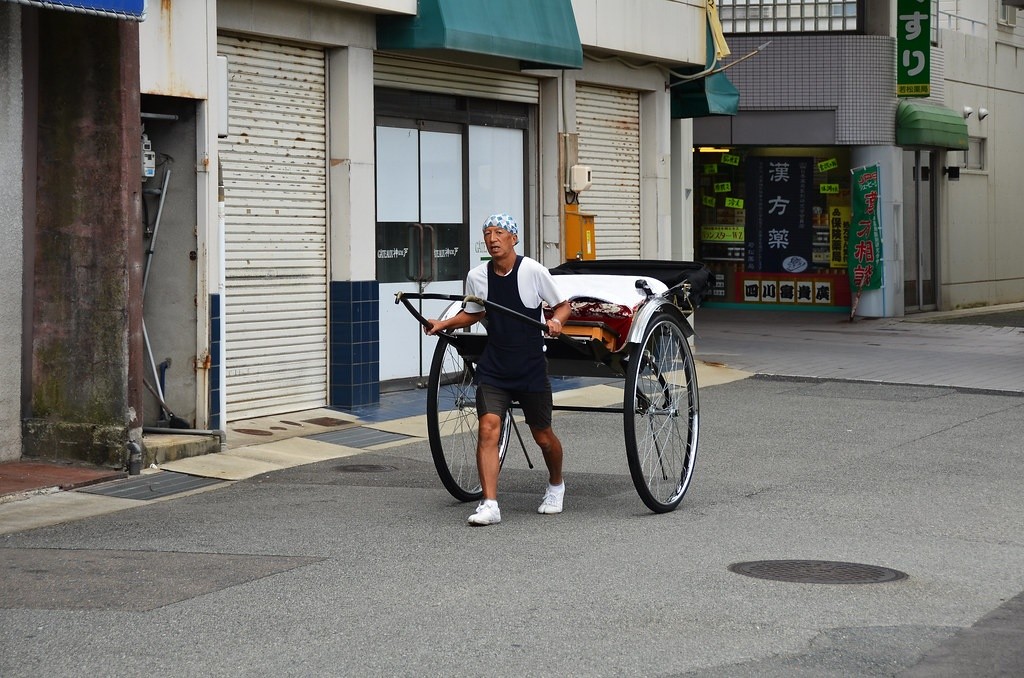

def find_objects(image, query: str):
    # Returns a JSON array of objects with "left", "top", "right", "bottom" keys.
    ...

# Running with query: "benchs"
[{"left": 539, "top": 274, "right": 670, "bottom": 351}]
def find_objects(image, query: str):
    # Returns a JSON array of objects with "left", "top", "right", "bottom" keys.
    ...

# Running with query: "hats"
[{"left": 482, "top": 213, "right": 518, "bottom": 234}]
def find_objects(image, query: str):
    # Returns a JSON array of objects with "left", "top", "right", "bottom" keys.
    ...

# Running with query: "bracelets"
[{"left": 551, "top": 317, "right": 562, "bottom": 325}]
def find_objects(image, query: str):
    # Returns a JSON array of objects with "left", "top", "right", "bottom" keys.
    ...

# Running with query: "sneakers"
[
  {"left": 537, "top": 478, "right": 565, "bottom": 513},
  {"left": 468, "top": 500, "right": 501, "bottom": 525}
]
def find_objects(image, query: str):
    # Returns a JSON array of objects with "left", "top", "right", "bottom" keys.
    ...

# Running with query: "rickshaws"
[{"left": 394, "top": 258, "right": 712, "bottom": 514}]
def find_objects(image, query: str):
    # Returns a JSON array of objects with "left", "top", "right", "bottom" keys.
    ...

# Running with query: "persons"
[{"left": 424, "top": 213, "right": 572, "bottom": 526}]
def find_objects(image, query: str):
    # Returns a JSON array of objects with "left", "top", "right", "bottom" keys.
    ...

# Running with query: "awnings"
[
  {"left": 896, "top": 98, "right": 970, "bottom": 152},
  {"left": 671, "top": 0, "right": 741, "bottom": 118},
  {"left": 376, "top": 0, "right": 585, "bottom": 71}
]
